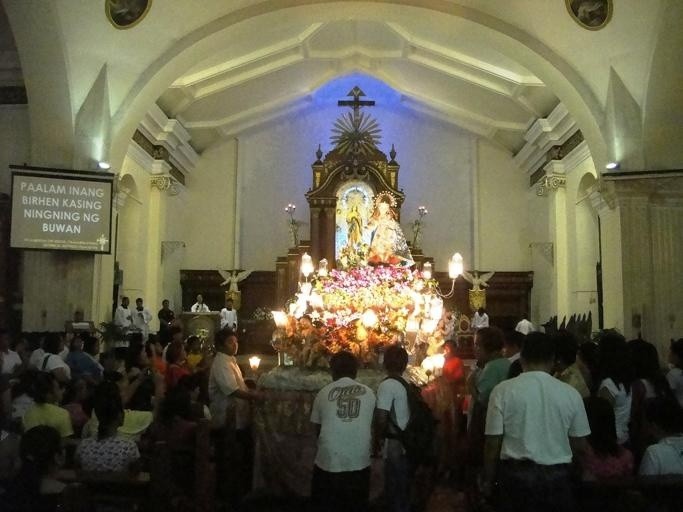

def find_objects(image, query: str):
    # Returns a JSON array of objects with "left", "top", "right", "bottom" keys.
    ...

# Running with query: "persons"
[
  {"left": 443, "top": 307, "right": 682, "bottom": 512},
  {"left": 0, "top": 294, "right": 267, "bottom": 512},
  {"left": 376, "top": 346, "right": 412, "bottom": 512},
  {"left": 310, "top": 350, "right": 377, "bottom": 512},
  {"left": 462, "top": 270, "right": 495, "bottom": 290},
  {"left": 346, "top": 205, "right": 363, "bottom": 245},
  {"left": 218, "top": 267, "right": 251, "bottom": 291}
]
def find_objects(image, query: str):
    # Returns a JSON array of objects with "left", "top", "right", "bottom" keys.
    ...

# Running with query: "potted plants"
[{"left": 96, "top": 322, "right": 124, "bottom": 352}]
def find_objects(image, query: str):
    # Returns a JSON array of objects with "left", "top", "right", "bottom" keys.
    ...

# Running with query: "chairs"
[{"left": 62, "top": 487, "right": 143, "bottom": 511}]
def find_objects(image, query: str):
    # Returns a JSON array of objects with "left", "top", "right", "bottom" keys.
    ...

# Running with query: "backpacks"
[{"left": 380, "top": 374, "right": 437, "bottom": 454}]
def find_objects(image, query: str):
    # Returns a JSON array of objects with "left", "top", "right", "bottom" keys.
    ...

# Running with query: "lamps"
[
  {"left": 422, "top": 356, "right": 433, "bottom": 383},
  {"left": 302, "top": 252, "right": 312, "bottom": 284},
  {"left": 421, "top": 261, "right": 432, "bottom": 284},
  {"left": 435, "top": 253, "right": 463, "bottom": 297}
]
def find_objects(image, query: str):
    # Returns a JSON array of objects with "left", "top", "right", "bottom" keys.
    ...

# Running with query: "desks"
[
  {"left": 56, "top": 470, "right": 150, "bottom": 482},
  {"left": 254, "top": 367, "right": 454, "bottom": 498}
]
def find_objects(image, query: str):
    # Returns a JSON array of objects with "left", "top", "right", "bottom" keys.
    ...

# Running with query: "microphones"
[{"left": 196, "top": 305, "right": 200, "bottom": 312}]
